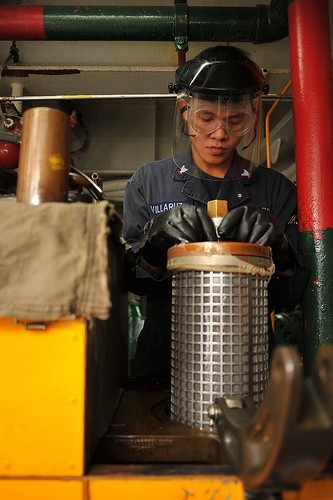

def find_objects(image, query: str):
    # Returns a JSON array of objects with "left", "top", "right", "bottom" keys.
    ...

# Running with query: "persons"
[{"left": 119, "top": 46, "right": 306, "bottom": 390}]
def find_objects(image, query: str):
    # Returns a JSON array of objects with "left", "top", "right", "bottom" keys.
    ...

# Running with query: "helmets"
[{"left": 168, "top": 45, "right": 270, "bottom": 103}]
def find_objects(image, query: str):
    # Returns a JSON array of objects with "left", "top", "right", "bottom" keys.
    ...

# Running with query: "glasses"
[{"left": 187, "top": 102, "right": 255, "bottom": 136}]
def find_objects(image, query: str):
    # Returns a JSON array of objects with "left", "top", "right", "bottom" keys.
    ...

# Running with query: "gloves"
[
  {"left": 136, "top": 204, "right": 218, "bottom": 280},
  {"left": 216, "top": 205, "right": 300, "bottom": 269}
]
